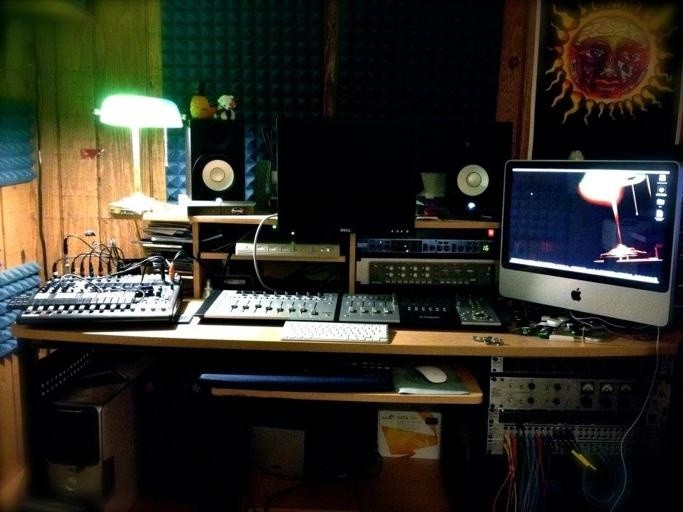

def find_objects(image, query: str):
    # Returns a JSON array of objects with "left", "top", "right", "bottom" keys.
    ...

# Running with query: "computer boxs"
[{"left": 43, "top": 368, "right": 147, "bottom": 505}]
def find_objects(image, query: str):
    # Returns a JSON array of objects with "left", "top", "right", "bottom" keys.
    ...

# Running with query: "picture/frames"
[{"left": 527, "top": 1, "right": 682, "bottom": 160}]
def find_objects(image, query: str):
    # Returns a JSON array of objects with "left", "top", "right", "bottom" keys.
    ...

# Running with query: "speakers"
[
  {"left": 446, "top": 123, "right": 512, "bottom": 220},
  {"left": 191, "top": 119, "right": 245, "bottom": 206}
]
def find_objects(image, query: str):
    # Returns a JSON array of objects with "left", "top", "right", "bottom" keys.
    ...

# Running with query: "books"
[{"left": 392, "top": 362, "right": 469, "bottom": 396}]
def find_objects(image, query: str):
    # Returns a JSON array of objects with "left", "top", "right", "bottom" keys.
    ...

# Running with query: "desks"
[{"left": 9, "top": 213, "right": 683, "bottom": 511}]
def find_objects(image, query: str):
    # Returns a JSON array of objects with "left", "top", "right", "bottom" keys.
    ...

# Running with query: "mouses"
[{"left": 415, "top": 365, "right": 448, "bottom": 384}]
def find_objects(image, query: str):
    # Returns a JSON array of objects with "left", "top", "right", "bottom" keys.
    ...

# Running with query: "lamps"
[{"left": 91, "top": 94, "right": 185, "bottom": 217}]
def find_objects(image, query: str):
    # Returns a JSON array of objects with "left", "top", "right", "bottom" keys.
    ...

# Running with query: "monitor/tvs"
[
  {"left": 277, "top": 117, "right": 416, "bottom": 238},
  {"left": 498, "top": 160, "right": 683, "bottom": 327}
]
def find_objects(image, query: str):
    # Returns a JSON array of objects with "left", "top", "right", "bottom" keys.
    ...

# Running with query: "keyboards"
[{"left": 281, "top": 320, "right": 389, "bottom": 344}]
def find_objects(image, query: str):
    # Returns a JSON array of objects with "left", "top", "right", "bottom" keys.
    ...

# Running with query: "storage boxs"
[
  {"left": 253, "top": 425, "right": 305, "bottom": 477},
  {"left": 376, "top": 408, "right": 442, "bottom": 461}
]
